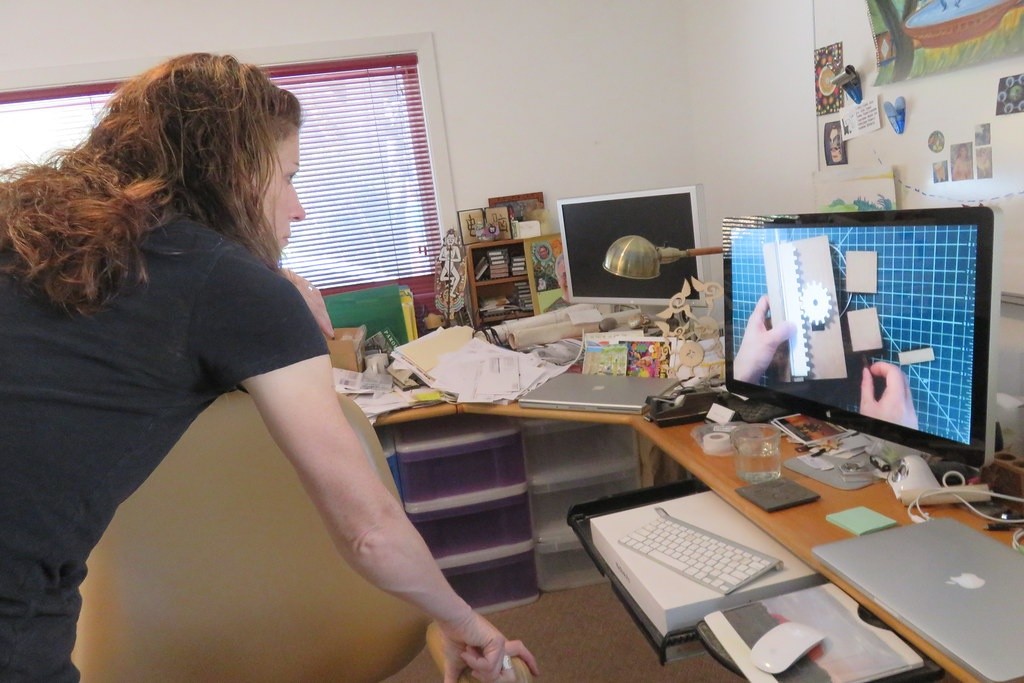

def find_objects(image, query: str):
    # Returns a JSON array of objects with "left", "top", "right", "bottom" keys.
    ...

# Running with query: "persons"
[
  {"left": 0, "top": 54, "right": 541, "bottom": 683},
  {"left": 733, "top": 294, "right": 918, "bottom": 431}
]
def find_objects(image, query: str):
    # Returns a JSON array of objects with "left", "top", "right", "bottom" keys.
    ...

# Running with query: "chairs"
[{"left": 72, "top": 389, "right": 532, "bottom": 683}]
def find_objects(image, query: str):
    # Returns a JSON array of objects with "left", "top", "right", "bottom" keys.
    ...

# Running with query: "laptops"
[
  {"left": 812, "top": 517, "right": 1024, "bottom": 683},
  {"left": 518, "top": 372, "right": 680, "bottom": 415}
]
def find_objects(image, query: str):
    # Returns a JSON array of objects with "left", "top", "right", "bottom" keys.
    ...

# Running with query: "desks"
[
  {"left": 458, "top": 398, "right": 1024, "bottom": 683},
  {"left": 371, "top": 401, "right": 456, "bottom": 428}
]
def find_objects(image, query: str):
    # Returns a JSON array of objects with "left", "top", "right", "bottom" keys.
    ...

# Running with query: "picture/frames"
[{"left": 488, "top": 192, "right": 544, "bottom": 222}]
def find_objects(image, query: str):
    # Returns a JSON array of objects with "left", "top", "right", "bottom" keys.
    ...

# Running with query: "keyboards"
[{"left": 619, "top": 514, "right": 784, "bottom": 595}]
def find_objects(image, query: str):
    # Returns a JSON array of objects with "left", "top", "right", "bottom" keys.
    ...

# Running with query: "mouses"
[{"left": 752, "top": 621, "right": 828, "bottom": 674}]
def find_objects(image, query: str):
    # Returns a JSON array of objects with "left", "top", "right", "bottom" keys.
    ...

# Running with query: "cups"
[
  {"left": 364, "top": 353, "right": 390, "bottom": 375},
  {"left": 730, "top": 422, "right": 782, "bottom": 485}
]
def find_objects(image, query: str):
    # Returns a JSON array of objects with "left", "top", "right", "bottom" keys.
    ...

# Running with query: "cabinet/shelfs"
[{"left": 463, "top": 238, "right": 535, "bottom": 329}]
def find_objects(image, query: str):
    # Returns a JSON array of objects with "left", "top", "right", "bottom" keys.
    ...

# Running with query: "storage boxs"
[
  {"left": 373, "top": 414, "right": 642, "bottom": 615},
  {"left": 323, "top": 324, "right": 367, "bottom": 374}
]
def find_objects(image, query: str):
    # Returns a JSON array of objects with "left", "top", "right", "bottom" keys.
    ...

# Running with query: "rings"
[{"left": 502, "top": 654, "right": 514, "bottom": 671}]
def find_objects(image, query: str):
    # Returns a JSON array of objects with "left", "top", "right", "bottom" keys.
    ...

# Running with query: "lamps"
[{"left": 601, "top": 235, "right": 722, "bottom": 280}]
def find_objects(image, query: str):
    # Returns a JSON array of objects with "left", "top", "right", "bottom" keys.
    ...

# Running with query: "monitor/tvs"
[
  {"left": 557, "top": 185, "right": 707, "bottom": 327},
  {"left": 723, "top": 207, "right": 996, "bottom": 491}
]
